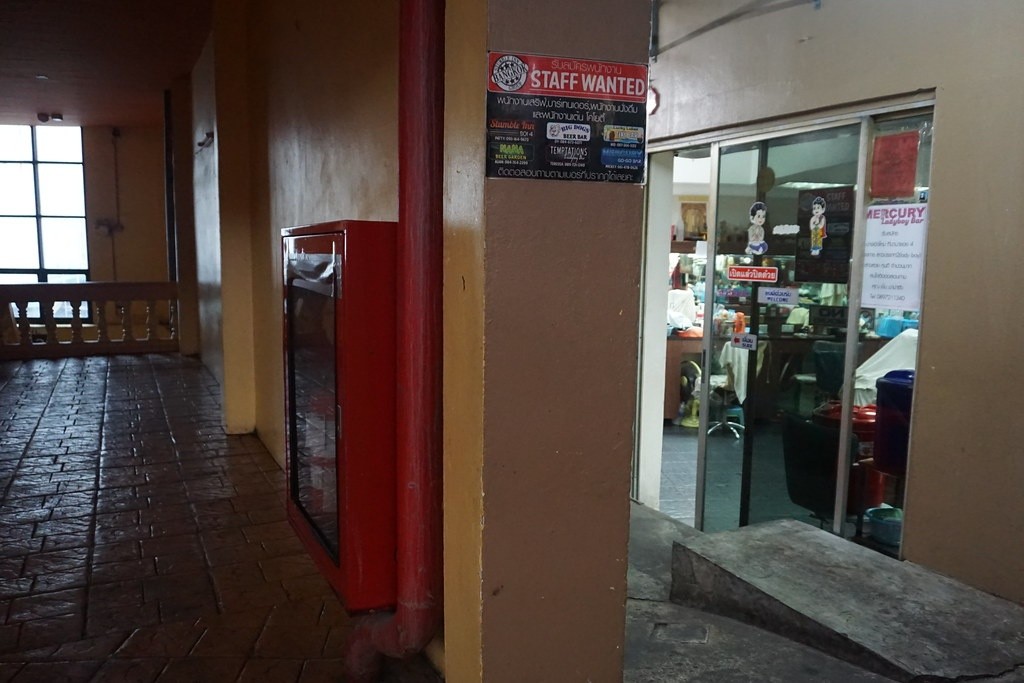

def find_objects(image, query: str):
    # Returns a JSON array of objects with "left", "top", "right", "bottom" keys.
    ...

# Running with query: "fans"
[{"left": 681, "top": 361, "right": 702, "bottom": 428}]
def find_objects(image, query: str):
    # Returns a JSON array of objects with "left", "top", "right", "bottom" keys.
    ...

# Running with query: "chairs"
[
  {"left": 777, "top": 412, "right": 883, "bottom": 536},
  {"left": 691, "top": 341, "right": 771, "bottom": 438},
  {"left": 791, "top": 340, "right": 865, "bottom": 417}
]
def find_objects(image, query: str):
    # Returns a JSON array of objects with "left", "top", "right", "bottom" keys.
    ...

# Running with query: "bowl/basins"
[{"left": 866, "top": 508, "right": 901, "bottom": 545}]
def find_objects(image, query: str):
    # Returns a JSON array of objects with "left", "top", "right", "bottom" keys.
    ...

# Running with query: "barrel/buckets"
[{"left": 814, "top": 400, "right": 884, "bottom": 517}]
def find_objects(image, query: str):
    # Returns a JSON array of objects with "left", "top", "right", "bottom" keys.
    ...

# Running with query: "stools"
[{"left": 713, "top": 405, "right": 744, "bottom": 425}]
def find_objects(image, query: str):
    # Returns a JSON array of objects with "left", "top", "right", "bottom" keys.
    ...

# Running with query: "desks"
[{"left": 665, "top": 331, "right": 894, "bottom": 430}]
